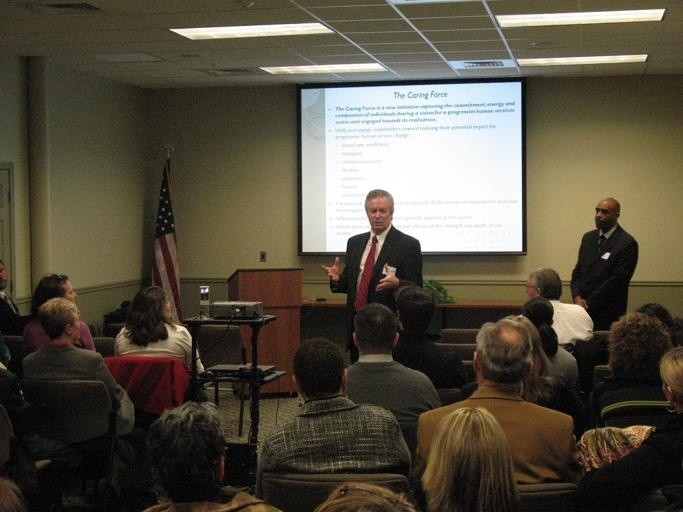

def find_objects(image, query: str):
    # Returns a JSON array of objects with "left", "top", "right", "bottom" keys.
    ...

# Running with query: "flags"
[{"left": 148, "top": 158, "right": 182, "bottom": 323}]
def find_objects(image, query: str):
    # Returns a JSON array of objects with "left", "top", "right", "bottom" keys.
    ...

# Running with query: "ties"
[
  {"left": 355, "top": 236, "right": 377, "bottom": 313},
  {"left": 593, "top": 236, "right": 604, "bottom": 258}
]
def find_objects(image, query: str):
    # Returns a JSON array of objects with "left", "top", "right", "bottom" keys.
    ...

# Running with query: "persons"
[
  {"left": 144, "top": 400, "right": 279, "bottom": 511},
  {"left": 24, "top": 298, "right": 145, "bottom": 473},
  {"left": 319, "top": 188, "right": 424, "bottom": 363},
  {"left": 387, "top": 283, "right": 475, "bottom": 391},
  {"left": 253, "top": 295, "right": 683, "bottom": 511},
  {"left": 517, "top": 268, "right": 595, "bottom": 345},
  {"left": 569, "top": 197, "right": 640, "bottom": 332},
  {"left": 20, "top": 272, "right": 97, "bottom": 352},
  {"left": 112, "top": 286, "right": 207, "bottom": 402},
  {"left": 0, "top": 257, "right": 32, "bottom": 337}
]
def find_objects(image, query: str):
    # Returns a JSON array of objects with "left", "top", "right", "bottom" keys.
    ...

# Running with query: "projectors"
[{"left": 213, "top": 301, "right": 264, "bottom": 319}]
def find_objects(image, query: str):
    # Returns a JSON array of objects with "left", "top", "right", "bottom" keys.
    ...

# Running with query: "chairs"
[
  {"left": 513, "top": 482, "right": 579, "bottom": 510},
  {"left": 18, "top": 379, "right": 113, "bottom": 446},
  {"left": 419, "top": 324, "right": 682, "bottom": 433},
  {"left": 2, "top": 335, "right": 27, "bottom": 374},
  {"left": 192, "top": 323, "right": 285, "bottom": 437},
  {"left": 660, "top": 484, "right": 681, "bottom": 510},
  {"left": 224, "top": 441, "right": 259, "bottom": 493},
  {"left": 261, "top": 473, "right": 415, "bottom": 511},
  {"left": 90, "top": 336, "right": 116, "bottom": 360}
]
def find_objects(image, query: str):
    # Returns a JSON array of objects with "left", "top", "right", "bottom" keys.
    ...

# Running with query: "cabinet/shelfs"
[{"left": 182, "top": 314, "right": 286, "bottom": 450}]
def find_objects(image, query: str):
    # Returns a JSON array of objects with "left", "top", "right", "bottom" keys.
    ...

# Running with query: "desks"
[{"left": 302, "top": 299, "right": 524, "bottom": 329}]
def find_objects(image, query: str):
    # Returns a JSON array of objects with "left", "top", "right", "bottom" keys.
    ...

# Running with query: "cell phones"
[{"left": 200, "top": 286, "right": 209, "bottom": 305}]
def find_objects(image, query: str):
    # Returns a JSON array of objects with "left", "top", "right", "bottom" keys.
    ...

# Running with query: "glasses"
[
  {"left": 524, "top": 283, "right": 537, "bottom": 289},
  {"left": 46, "top": 272, "right": 66, "bottom": 285}
]
